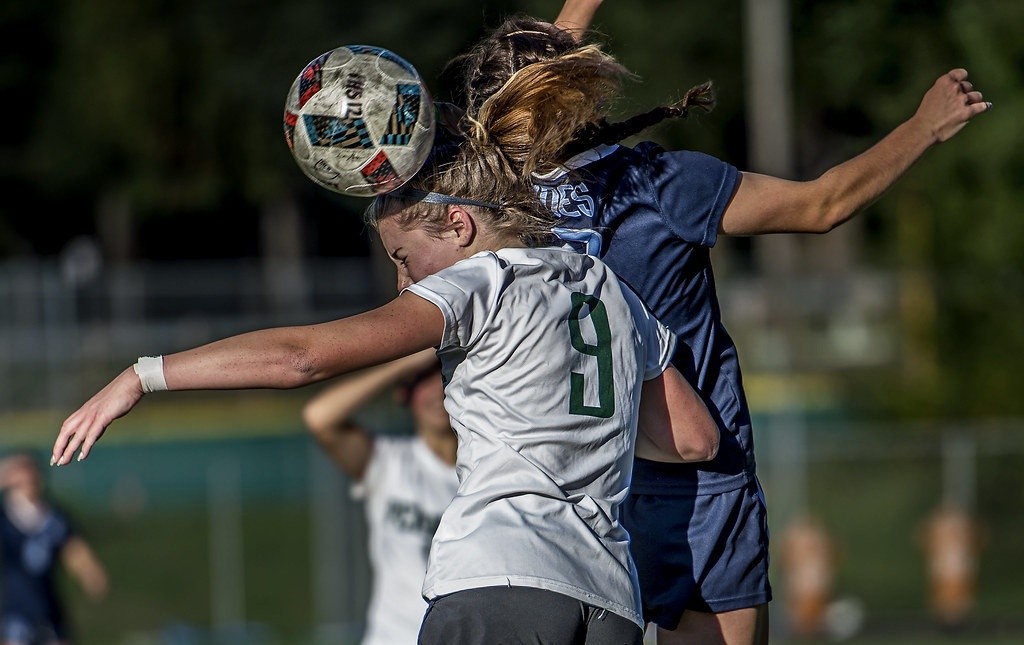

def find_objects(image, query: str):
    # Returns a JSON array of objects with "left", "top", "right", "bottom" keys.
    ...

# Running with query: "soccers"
[{"left": 282, "top": 44, "right": 439, "bottom": 200}]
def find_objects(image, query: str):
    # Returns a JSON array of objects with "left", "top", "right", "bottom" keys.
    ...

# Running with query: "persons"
[
  {"left": 464, "top": 20, "right": 994, "bottom": 644},
  {"left": 0, "top": 452, "right": 106, "bottom": 645},
  {"left": 303, "top": 346, "right": 459, "bottom": 645},
  {"left": 52, "top": 45, "right": 720, "bottom": 645}
]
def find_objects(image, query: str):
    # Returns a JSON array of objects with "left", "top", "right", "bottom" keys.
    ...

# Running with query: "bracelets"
[{"left": 133, "top": 354, "right": 167, "bottom": 393}]
